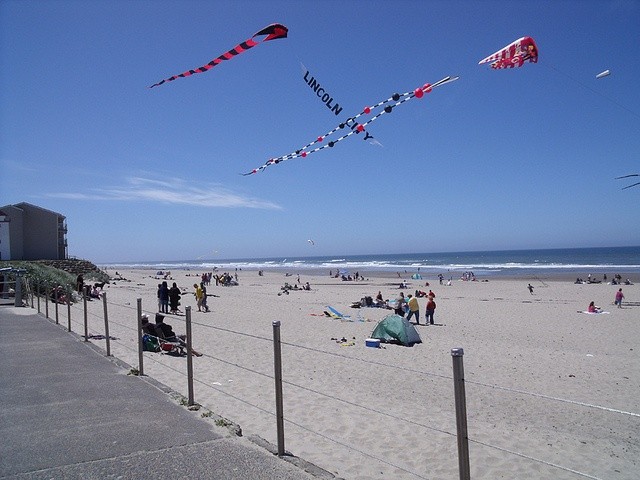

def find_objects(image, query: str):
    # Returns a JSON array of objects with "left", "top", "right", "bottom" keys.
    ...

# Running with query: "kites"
[
  {"left": 238, "top": 35, "right": 539, "bottom": 177},
  {"left": 147, "top": 22, "right": 384, "bottom": 149},
  {"left": 594, "top": 70, "right": 611, "bottom": 82},
  {"left": 305, "top": 305, "right": 352, "bottom": 322}
]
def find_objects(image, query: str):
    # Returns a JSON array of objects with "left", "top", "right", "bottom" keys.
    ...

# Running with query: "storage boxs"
[{"left": 365, "top": 338, "right": 380, "bottom": 347}]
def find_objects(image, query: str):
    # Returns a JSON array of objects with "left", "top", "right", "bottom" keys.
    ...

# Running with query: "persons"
[
  {"left": 76, "top": 272, "right": 85, "bottom": 294},
  {"left": 446, "top": 278, "right": 452, "bottom": 286},
  {"left": 405, "top": 293, "right": 419, "bottom": 324},
  {"left": 438, "top": 273, "right": 444, "bottom": 285},
  {"left": 199, "top": 282, "right": 209, "bottom": 311},
  {"left": 360, "top": 289, "right": 385, "bottom": 308},
  {"left": 192, "top": 283, "right": 204, "bottom": 312},
  {"left": 415, "top": 289, "right": 428, "bottom": 298},
  {"left": 141, "top": 313, "right": 155, "bottom": 357},
  {"left": 302, "top": 281, "right": 311, "bottom": 290},
  {"left": 397, "top": 271, "right": 402, "bottom": 278},
  {"left": 202, "top": 271, "right": 213, "bottom": 287},
  {"left": 159, "top": 281, "right": 171, "bottom": 314},
  {"left": 463, "top": 271, "right": 477, "bottom": 281},
  {"left": 527, "top": 283, "right": 534, "bottom": 295},
  {"left": 424, "top": 296, "right": 436, "bottom": 326},
  {"left": 429, "top": 290, "right": 435, "bottom": 297},
  {"left": 82, "top": 284, "right": 102, "bottom": 301},
  {"left": 281, "top": 282, "right": 292, "bottom": 293},
  {"left": 297, "top": 274, "right": 301, "bottom": 284},
  {"left": 156, "top": 284, "right": 162, "bottom": 312},
  {"left": 342, "top": 272, "right": 364, "bottom": 280},
  {"left": 155, "top": 270, "right": 172, "bottom": 280},
  {"left": 155, "top": 312, "right": 203, "bottom": 358},
  {"left": 589, "top": 301, "right": 598, "bottom": 313},
  {"left": 168, "top": 282, "right": 182, "bottom": 315},
  {"left": 398, "top": 283, "right": 408, "bottom": 289},
  {"left": 615, "top": 287, "right": 626, "bottom": 309},
  {"left": 394, "top": 289, "right": 407, "bottom": 316},
  {"left": 329, "top": 268, "right": 341, "bottom": 278},
  {"left": 50, "top": 285, "right": 74, "bottom": 305},
  {"left": 575, "top": 272, "right": 634, "bottom": 285},
  {"left": 185, "top": 273, "right": 201, "bottom": 277},
  {"left": 213, "top": 272, "right": 240, "bottom": 287}
]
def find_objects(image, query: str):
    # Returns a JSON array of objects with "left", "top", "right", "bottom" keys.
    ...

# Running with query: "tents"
[{"left": 369, "top": 314, "right": 422, "bottom": 347}]
